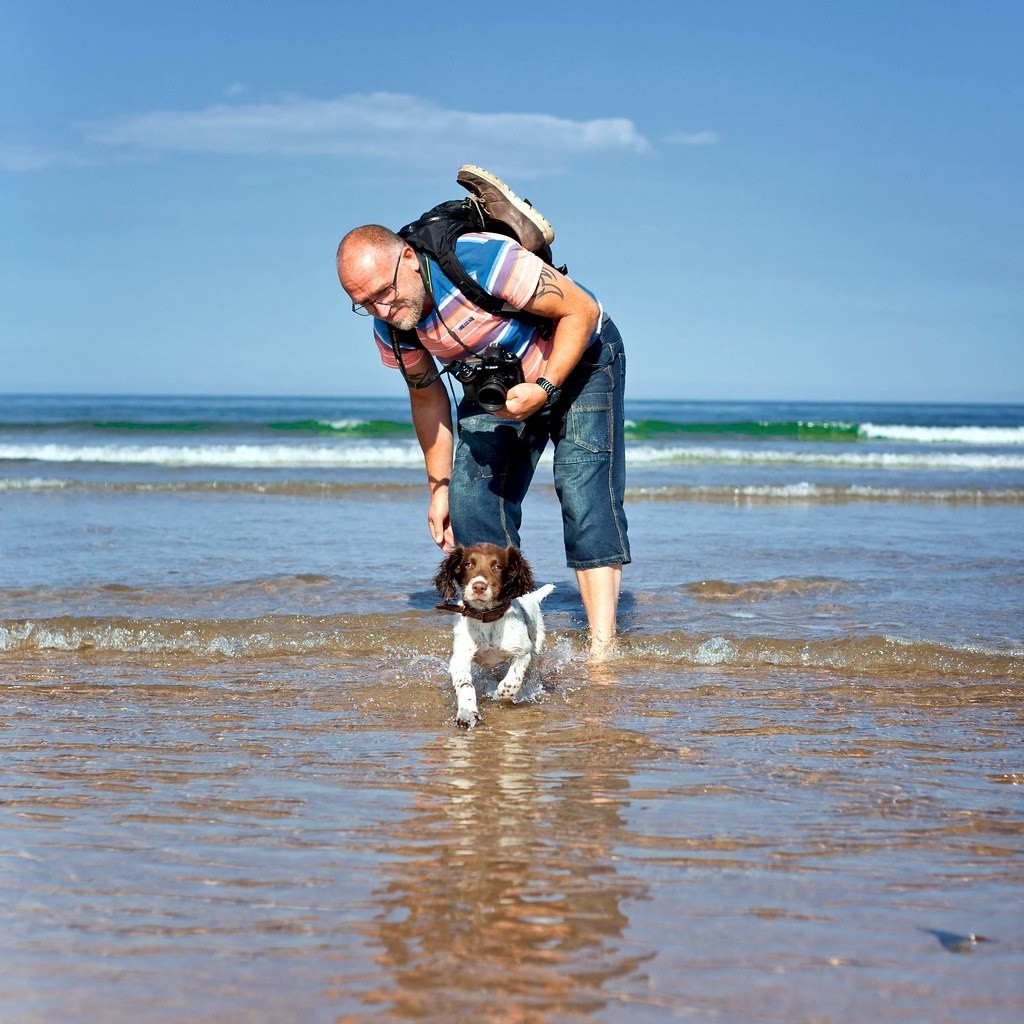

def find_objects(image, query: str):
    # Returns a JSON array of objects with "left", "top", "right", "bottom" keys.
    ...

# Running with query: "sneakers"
[{"left": 456, "top": 164, "right": 556, "bottom": 252}]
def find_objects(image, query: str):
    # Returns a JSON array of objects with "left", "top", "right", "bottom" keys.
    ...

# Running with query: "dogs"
[{"left": 432, "top": 543, "right": 557, "bottom": 729}]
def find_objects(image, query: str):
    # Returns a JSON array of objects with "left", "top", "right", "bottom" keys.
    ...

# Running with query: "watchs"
[{"left": 536, "top": 377, "right": 562, "bottom": 405}]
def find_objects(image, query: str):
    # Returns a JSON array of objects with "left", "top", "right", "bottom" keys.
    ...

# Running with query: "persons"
[{"left": 335, "top": 200, "right": 629, "bottom": 649}]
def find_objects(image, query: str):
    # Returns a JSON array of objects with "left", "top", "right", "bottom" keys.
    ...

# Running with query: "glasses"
[{"left": 351, "top": 243, "right": 408, "bottom": 317}]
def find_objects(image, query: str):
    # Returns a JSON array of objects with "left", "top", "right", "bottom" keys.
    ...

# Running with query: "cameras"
[{"left": 460, "top": 344, "right": 525, "bottom": 412}]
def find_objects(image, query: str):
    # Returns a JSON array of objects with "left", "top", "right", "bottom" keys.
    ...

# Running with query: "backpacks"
[{"left": 386, "top": 198, "right": 571, "bottom": 349}]
